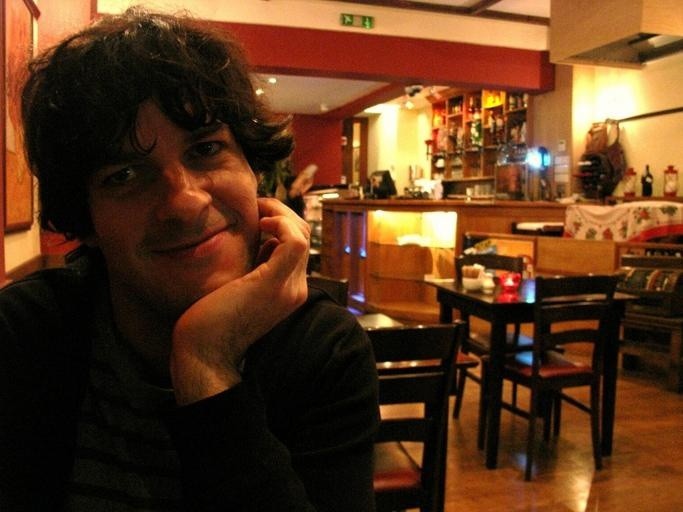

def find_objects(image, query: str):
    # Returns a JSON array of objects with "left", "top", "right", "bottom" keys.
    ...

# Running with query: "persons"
[{"left": 1, "top": 4, "right": 379, "bottom": 511}]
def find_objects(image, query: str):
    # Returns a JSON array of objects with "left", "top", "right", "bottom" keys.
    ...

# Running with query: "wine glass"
[{"left": 466, "top": 187, "right": 473, "bottom": 202}]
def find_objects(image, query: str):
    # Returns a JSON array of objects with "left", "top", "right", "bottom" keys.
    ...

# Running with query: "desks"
[{"left": 425, "top": 278, "right": 639, "bottom": 470}]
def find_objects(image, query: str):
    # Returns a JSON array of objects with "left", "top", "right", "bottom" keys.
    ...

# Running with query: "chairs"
[
  {"left": 453, "top": 253, "right": 565, "bottom": 435},
  {"left": 364, "top": 319, "right": 464, "bottom": 512},
  {"left": 478, "top": 274, "right": 619, "bottom": 481}
]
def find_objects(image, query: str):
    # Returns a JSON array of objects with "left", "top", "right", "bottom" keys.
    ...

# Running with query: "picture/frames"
[{"left": 3, "top": 1, "right": 41, "bottom": 233}]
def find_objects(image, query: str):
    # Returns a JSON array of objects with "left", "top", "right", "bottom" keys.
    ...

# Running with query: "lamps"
[{"left": 526, "top": 146, "right": 552, "bottom": 200}]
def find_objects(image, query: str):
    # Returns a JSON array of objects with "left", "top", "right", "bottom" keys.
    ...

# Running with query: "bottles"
[{"left": 641, "top": 164, "right": 652, "bottom": 196}]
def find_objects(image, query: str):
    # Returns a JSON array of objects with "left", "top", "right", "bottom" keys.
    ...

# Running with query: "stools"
[{"left": 512, "top": 218, "right": 564, "bottom": 237}]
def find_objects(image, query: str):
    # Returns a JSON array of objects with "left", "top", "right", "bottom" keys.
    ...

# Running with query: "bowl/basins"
[{"left": 462, "top": 277, "right": 481, "bottom": 291}]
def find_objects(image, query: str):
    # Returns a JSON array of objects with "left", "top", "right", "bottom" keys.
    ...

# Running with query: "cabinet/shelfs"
[{"left": 425, "top": 87, "right": 534, "bottom": 199}]
